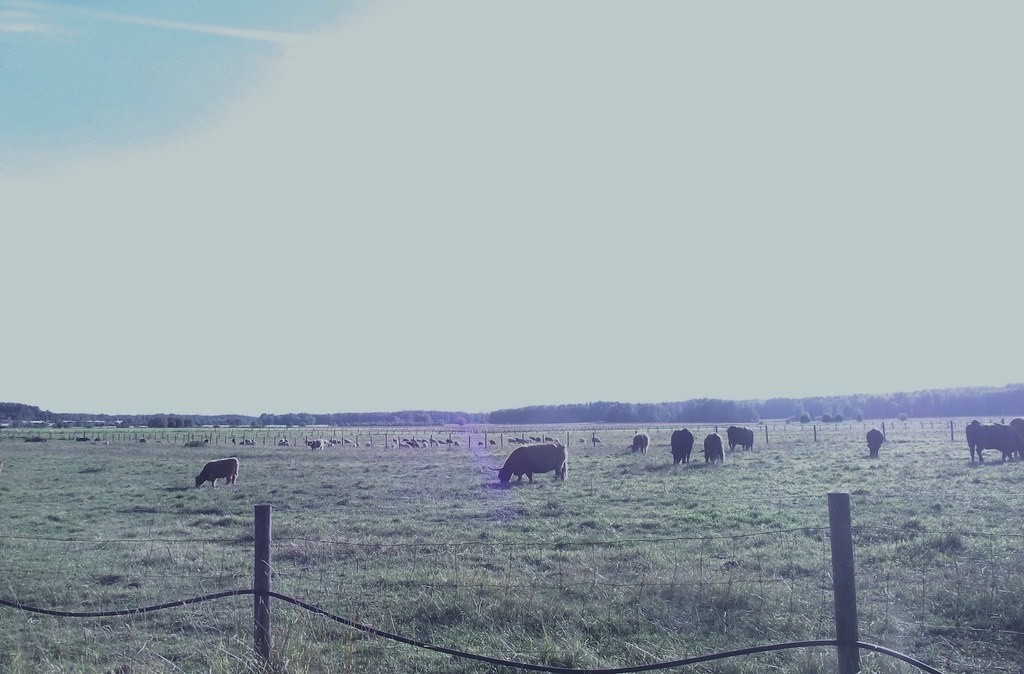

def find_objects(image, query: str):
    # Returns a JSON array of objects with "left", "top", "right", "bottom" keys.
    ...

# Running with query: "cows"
[
  {"left": 627, "top": 432, "right": 650, "bottom": 453},
  {"left": 278, "top": 438, "right": 289, "bottom": 447},
  {"left": 966, "top": 420, "right": 1024, "bottom": 464},
  {"left": 726, "top": 426, "right": 754, "bottom": 451},
  {"left": 507, "top": 437, "right": 553, "bottom": 443},
  {"left": 195, "top": 456, "right": 239, "bottom": 486},
  {"left": 1009, "top": 418, "right": 1024, "bottom": 439},
  {"left": 866, "top": 429, "right": 884, "bottom": 458},
  {"left": 392, "top": 438, "right": 459, "bottom": 448},
  {"left": 490, "top": 440, "right": 496, "bottom": 444},
  {"left": 579, "top": 438, "right": 586, "bottom": 443},
  {"left": 365, "top": 443, "right": 371, "bottom": 446},
  {"left": 24, "top": 437, "right": 162, "bottom": 443},
  {"left": 670, "top": 428, "right": 694, "bottom": 463},
  {"left": 592, "top": 437, "right": 601, "bottom": 443},
  {"left": 184, "top": 437, "right": 256, "bottom": 447},
  {"left": 306, "top": 439, "right": 352, "bottom": 450},
  {"left": 488, "top": 443, "right": 569, "bottom": 483},
  {"left": 698, "top": 433, "right": 724, "bottom": 464},
  {"left": 478, "top": 441, "right": 484, "bottom": 445}
]
